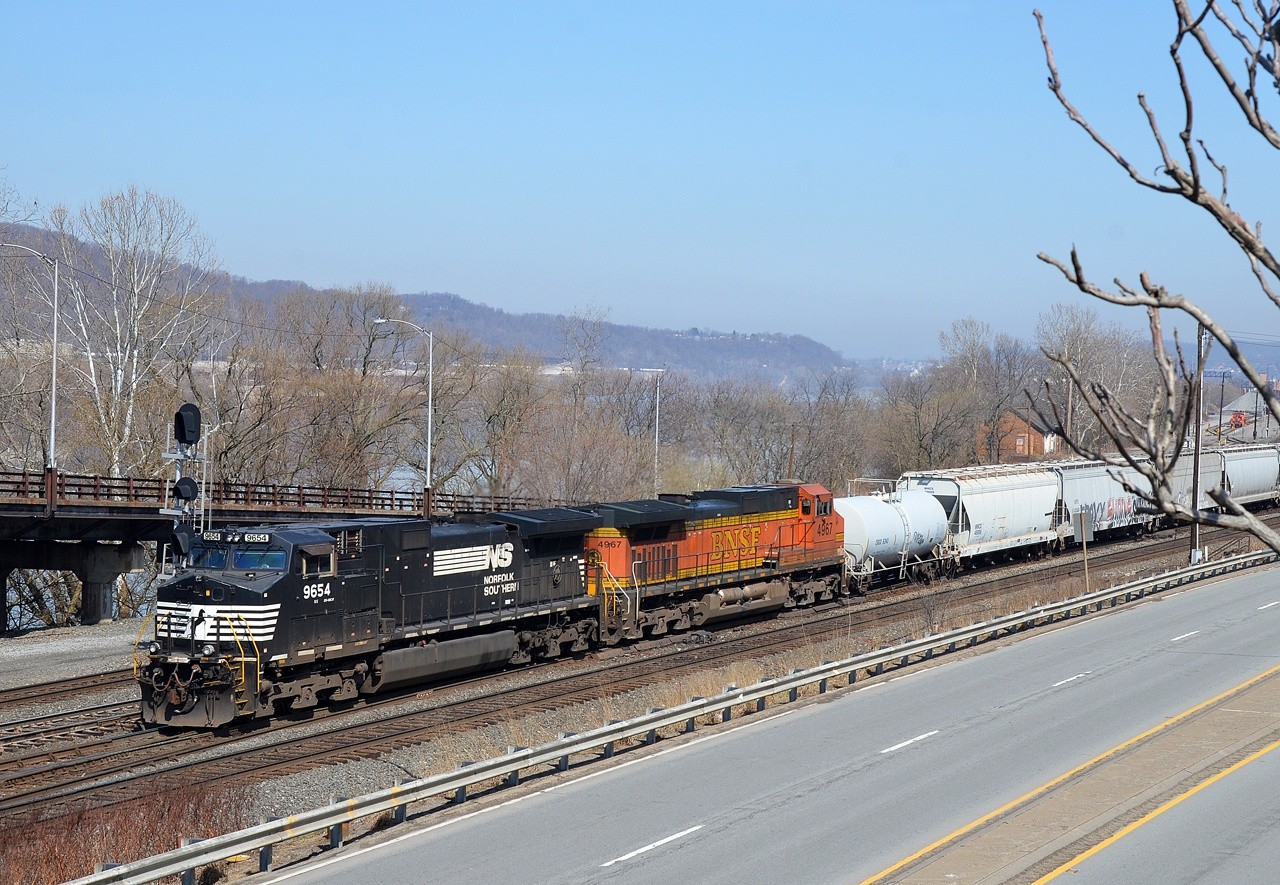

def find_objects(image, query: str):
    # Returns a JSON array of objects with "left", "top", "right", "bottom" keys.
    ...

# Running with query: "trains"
[{"left": 130, "top": 441, "right": 1280, "bottom": 740}]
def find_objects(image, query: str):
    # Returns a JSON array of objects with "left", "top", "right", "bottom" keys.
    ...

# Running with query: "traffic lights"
[
  {"left": 170, "top": 524, "right": 195, "bottom": 556},
  {"left": 173, "top": 477, "right": 198, "bottom": 500},
  {"left": 174, "top": 403, "right": 201, "bottom": 444}
]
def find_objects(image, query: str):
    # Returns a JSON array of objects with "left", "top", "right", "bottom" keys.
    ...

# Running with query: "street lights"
[{"left": 373, "top": 318, "right": 434, "bottom": 488}]
